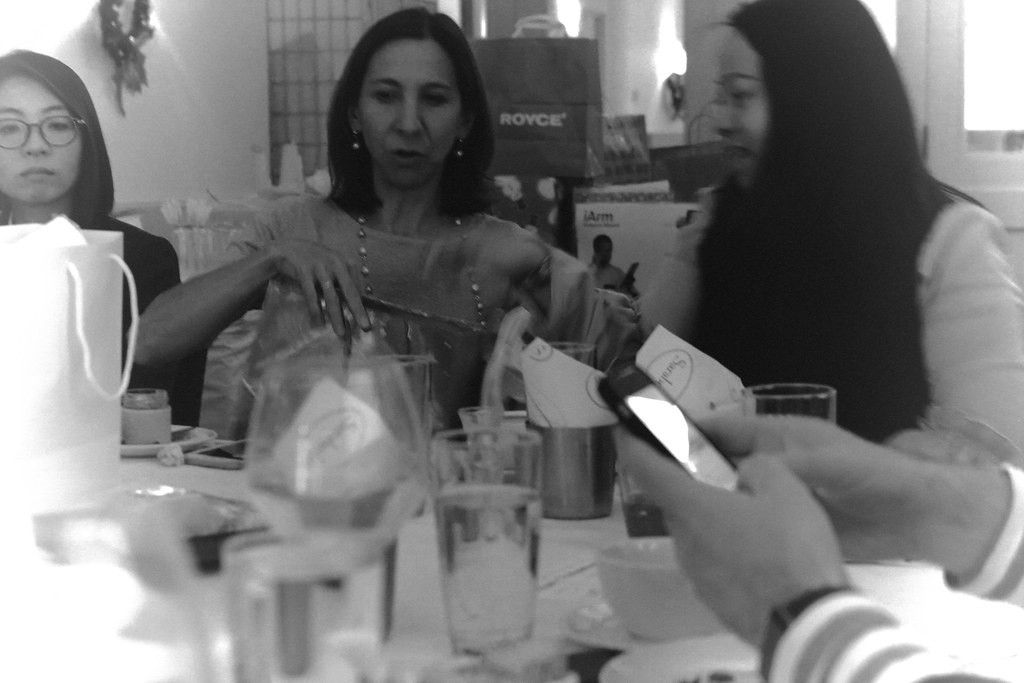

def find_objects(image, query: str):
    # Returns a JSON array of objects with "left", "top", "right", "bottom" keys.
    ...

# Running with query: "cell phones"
[
  {"left": 597, "top": 362, "right": 740, "bottom": 493},
  {"left": 185, "top": 439, "right": 247, "bottom": 470}
]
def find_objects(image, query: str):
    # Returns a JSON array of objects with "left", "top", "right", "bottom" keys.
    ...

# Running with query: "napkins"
[{"left": 520, "top": 339, "right": 618, "bottom": 429}]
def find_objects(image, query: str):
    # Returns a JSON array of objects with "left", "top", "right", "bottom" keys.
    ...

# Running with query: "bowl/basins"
[{"left": 596, "top": 537, "right": 720, "bottom": 642}]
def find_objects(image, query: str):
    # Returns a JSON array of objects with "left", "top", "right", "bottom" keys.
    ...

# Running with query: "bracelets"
[{"left": 758, "top": 586, "right": 848, "bottom": 680}]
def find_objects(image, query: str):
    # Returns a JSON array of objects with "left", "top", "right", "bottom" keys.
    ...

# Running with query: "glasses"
[{"left": 0, "top": 115, "right": 86, "bottom": 150}]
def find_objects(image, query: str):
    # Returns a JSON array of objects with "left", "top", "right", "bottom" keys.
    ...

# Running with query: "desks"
[{"left": 0, "top": 437, "right": 934, "bottom": 683}]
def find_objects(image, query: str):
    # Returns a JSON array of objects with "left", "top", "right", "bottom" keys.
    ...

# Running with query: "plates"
[{"left": 121, "top": 424, "right": 218, "bottom": 457}]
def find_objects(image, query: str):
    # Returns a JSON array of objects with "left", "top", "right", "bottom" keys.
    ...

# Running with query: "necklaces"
[{"left": 357, "top": 213, "right": 486, "bottom": 340}]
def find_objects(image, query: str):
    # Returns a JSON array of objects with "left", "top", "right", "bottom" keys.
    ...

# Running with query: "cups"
[
  {"left": 121, "top": 405, "right": 171, "bottom": 444},
  {"left": 433, "top": 429, "right": 541, "bottom": 650},
  {"left": 751, "top": 385, "right": 837, "bottom": 426},
  {"left": 221, "top": 533, "right": 364, "bottom": 683},
  {"left": 525, "top": 418, "right": 618, "bottom": 519},
  {"left": 460, "top": 406, "right": 481, "bottom": 427},
  {"left": 616, "top": 462, "right": 671, "bottom": 537},
  {"left": 548, "top": 341, "right": 594, "bottom": 367}
]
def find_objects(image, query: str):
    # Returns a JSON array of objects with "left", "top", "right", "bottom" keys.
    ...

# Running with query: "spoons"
[{"left": 121, "top": 427, "right": 197, "bottom": 444}]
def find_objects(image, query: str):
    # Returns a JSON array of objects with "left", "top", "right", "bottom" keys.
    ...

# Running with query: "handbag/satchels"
[{"left": 0, "top": 222, "right": 139, "bottom": 516}]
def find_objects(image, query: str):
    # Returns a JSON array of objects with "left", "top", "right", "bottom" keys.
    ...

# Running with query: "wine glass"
[{"left": 241, "top": 356, "right": 431, "bottom": 677}]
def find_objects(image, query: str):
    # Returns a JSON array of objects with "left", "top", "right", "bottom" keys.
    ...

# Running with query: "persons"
[
  {"left": 127, "top": 7, "right": 640, "bottom": 453},
  {"left": 586, "top": 234, "right": 635, "bottom": 298},
  {"left": 641, "top": 0, "right": 1024, "bottom": 472},
  {"left": 0, "top": 49, "right": 205, "bottom": 428},
  {"left": 613, "top": 415, "right": 1024, "bottom": 683}
]
